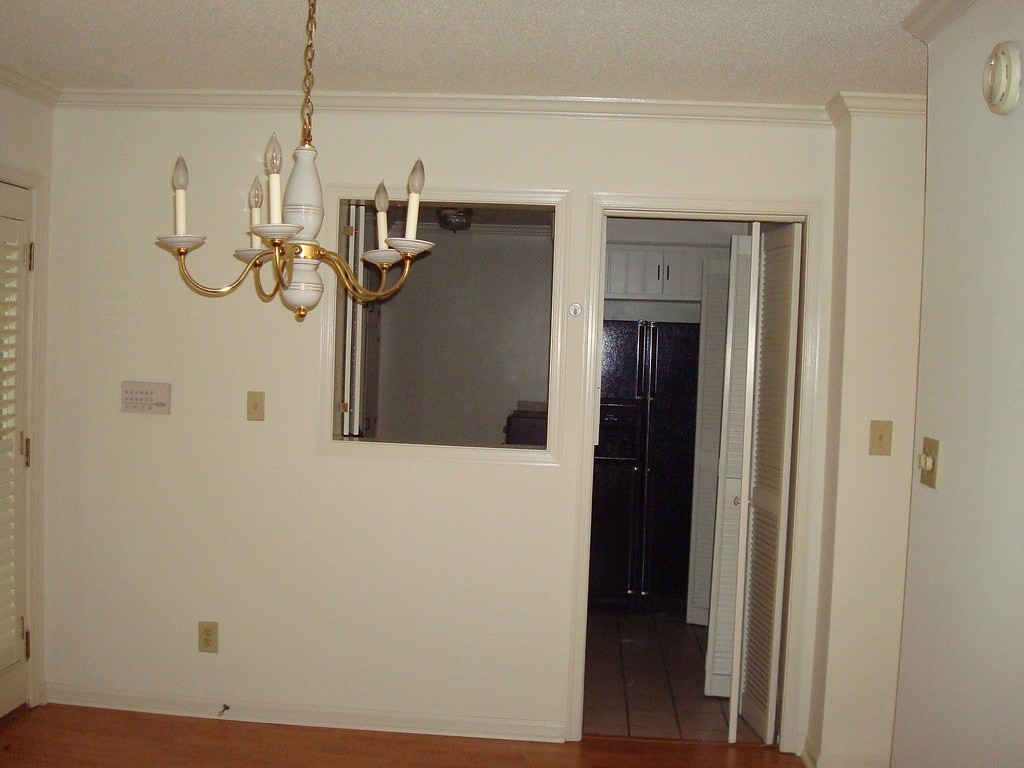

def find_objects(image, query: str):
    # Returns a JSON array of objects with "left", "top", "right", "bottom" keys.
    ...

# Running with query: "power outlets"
[
  {"left": 920, "top": 437, "right": 941, "bottom": 490},
  {"left": 198, "top": 621, "right": 219, "bottom": 654}
]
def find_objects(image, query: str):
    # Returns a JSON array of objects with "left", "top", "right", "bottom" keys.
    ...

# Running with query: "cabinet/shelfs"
[
  {"left": 603, "top": 215, "right": 749, "bottom": 324},
  {"left": 585, "top": 319, "right": 700, "bottom": 621}
]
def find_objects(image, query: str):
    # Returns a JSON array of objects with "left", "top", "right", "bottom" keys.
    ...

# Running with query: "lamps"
[
  {"left": 436, "top": 207, "right": 474, "bottom": 235},
  {"left": 156, "top": 0, "right": 440, "bottom": 320}
]
